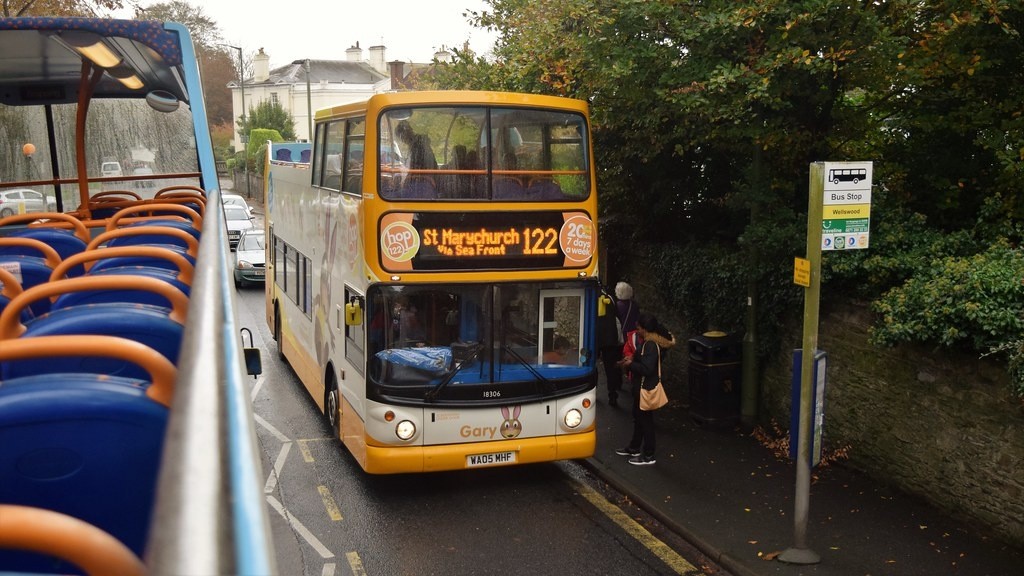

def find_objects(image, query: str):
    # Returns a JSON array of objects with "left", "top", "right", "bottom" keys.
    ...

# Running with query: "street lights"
[
  {"left": 292, "top": 59, "right": 313, "bottom": 142},
  {"left": 215, "top": 42, "right": 251, "bottom": 198}
]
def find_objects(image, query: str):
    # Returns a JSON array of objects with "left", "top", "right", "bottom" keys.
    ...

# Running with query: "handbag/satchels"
[
  {"left": 639, "top": 382, "right": 669, "bottom": 411},
  {"left": 616, "top": 317, "right": 625, "bottom": 348}
]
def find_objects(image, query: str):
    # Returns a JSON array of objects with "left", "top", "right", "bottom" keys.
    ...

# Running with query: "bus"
[
  {"left": 0, "top": 15, "right": 276, "bottom": 576},
  {"left": 263, "top": 89, "right": 613, "bottom": 480}
]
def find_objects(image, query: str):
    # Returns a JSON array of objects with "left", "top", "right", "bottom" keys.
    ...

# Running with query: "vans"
[{"left": 101, "top": 162, "right": 124, "bottom": 184}]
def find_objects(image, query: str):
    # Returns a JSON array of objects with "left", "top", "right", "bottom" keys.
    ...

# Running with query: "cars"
[
  {"left": 132, "top": 167, "right": 156, "bottom": 188},
  {"left": 230, "top": 229, "right": 266, "bottom": 289},
  {"left": 222, "top": 204, "right": 256, "bottom": 251},
  {"left": 222, "top": 194, "right": 254, "bottom": 221}
]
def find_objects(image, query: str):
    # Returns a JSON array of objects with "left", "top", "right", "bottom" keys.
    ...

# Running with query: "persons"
[
  {"left": 614, "top": 311, "right": 677, "bottom": 467},
  {"left": 393, "top": 120, "right": 530, "bottom": 190},
  {"left": 599, "top": 284, "right": 624, "bottom": 409},
  {"left": 554, "top": 336, "right": 570, "bottom": 355},
  {"left": 368, "top": 291, "right": 431, "bottom": 349},
  {"left": 621, "top": 318, "right": 645, "bottom": 383},
  {"left": 615, "top": 280, "right": 642, "bottom": 373}
]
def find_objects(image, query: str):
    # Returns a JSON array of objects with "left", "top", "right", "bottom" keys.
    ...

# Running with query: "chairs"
[
  {"left": 0, "top": 186, "right": 208, "bottom": 576},
  {"left": 321, "top": 164, "right": 562, "bottom": 202}
]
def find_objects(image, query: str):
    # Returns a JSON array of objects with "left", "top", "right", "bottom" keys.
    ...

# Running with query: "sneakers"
[
  {"left": 628, "top": 455, "right": 656, "bottom": 465},
  {"left": 615, "top": 447, "right": 641, "bottom": 457}
]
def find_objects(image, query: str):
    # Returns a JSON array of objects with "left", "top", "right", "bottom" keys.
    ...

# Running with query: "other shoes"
[{"left": 608, "top": 397, "right": 617, "bottom": 406}]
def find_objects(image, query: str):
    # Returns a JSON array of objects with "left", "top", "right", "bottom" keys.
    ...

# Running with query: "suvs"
[{"left": 0, "top": 189, "right": 57, "bottom": 219}]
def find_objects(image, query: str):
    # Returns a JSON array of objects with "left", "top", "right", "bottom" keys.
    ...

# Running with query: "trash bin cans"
[{"left": 685, "top": 331, "right": 744, "bottom": 431}]
